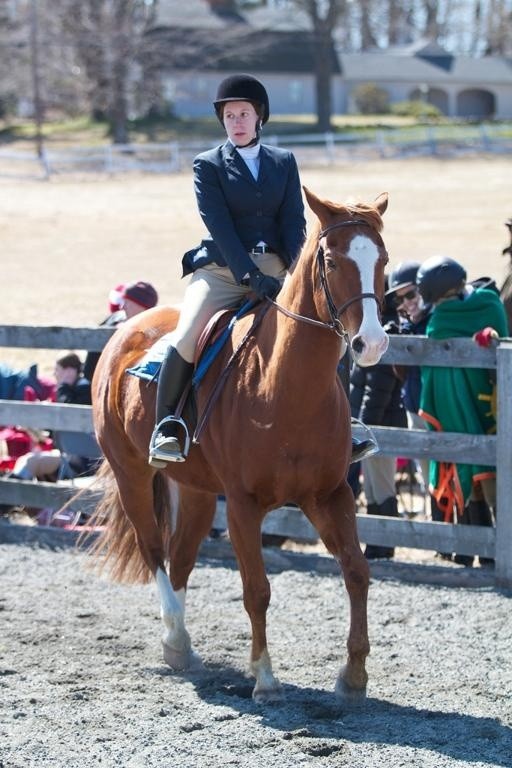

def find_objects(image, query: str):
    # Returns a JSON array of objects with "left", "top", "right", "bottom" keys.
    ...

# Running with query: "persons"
[
  {"left": 259, "top": 257, "right": 512, "bottom": 567},
  {"left": 2, "top": 281, "right": 158, "bottom": 517},
  {"left": 148, "top": 75, "right": 307, "bottom": 470}
]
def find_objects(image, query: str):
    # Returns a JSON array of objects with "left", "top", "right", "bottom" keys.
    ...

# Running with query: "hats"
[{"left": 123, "top": 283, "right": 157, "bottom": 308}]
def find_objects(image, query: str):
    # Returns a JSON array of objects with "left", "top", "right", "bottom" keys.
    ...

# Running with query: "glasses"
[{"left": 388, "top": 288, "right": 417, "bottom": 308}]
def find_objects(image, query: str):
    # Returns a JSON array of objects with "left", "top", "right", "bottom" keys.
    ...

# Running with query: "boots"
[
  {"left": 148, "top": 346, "right": 196, "bottom": 467},
  {"left": 361, "top": 495, "right": 497, "bottom": 568}
]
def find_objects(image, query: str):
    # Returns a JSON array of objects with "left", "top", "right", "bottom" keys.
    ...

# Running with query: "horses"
[{"left": 69, "top": 185, "right": 390, "bottom": 709}]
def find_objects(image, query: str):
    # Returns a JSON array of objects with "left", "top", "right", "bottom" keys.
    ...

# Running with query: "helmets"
[
  {"left": 412, "top": 258, "right": 466, "bottom": 311},
  {"left": 213, "top": 74, "right": 270, "bottom": 126},
  {"left": 382, "top": 257, "right": 419, "bottom": 298}
]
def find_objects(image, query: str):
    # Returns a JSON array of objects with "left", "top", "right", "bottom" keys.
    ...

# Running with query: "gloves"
[{"left": 249, "top": 271, "right": 280, "bottom": 300}]
